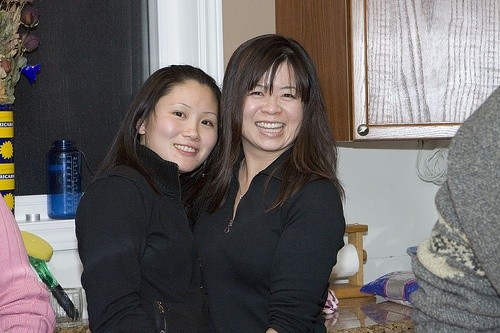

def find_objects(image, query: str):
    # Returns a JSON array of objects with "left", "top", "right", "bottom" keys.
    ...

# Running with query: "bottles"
[{"left": 46, "top": 139, "right": 82, "bottom": 219}]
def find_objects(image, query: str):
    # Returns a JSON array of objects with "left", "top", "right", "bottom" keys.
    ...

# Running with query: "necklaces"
[{"left": 238, "top": 157, "right": 245, "bottom": 199}]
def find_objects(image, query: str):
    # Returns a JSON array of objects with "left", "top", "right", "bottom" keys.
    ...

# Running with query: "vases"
[{"left": 0, "top": 103, "right": 15, "bottom": 217}]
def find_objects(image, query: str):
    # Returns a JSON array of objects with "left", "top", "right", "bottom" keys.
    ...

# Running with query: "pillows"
[
  {"left": 361, "top": 301, "right": 417, "bottom": 325},
  {"left": 360, "top": 272, "right": 420, "bottom": 301}
]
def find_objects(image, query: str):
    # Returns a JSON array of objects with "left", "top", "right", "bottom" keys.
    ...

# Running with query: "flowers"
[{"left": 0, "top": 0, "right": 44, "bottom": 103}]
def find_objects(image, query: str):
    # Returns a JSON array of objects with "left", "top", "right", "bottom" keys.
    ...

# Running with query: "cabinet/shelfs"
[{"left": 276, "top": 0, "right": 500, "bottom": 142}]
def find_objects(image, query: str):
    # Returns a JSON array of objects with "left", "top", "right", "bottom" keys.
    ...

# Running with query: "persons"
[
  {"left": 191, "top": 33, "right": 346, "bottom": 333},
  {"left": 0, "top": 192, "right": 57, "bottom": 333},
  {"left": 410, "top": 86, "right": 500, "bottom": 333},
  {"left": 74, "top": 65, "right": 221, "bottom": 333}
]
